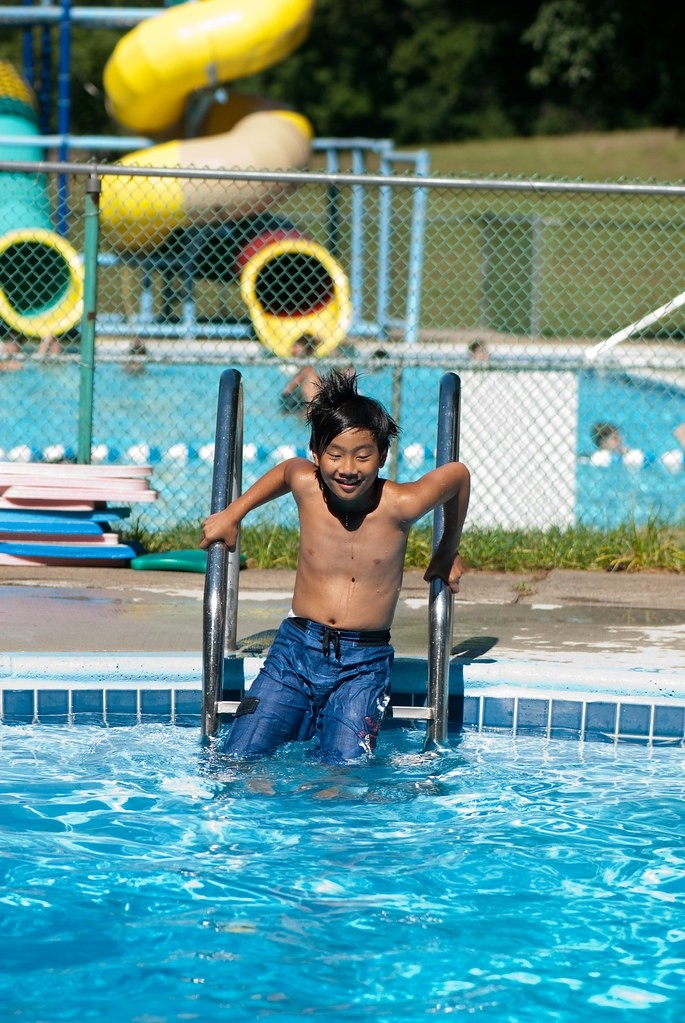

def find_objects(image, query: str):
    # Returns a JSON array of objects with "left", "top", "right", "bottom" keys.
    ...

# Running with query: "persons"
[
  {"left": 468, "top": 339, "right": 488, "bottom": 360},
  {"left": 284, "top": 333, "right": 356, "bottom": 410},
  {"left": 201, "top": 364, "right": 472, "bottom": 761},
  {"left": 368, "top": 349, "right": 390, "bottom": 371},
  {"left": 127, "top": 346, "right": 148, "bottom": 372},
  {"left": 591, "top": 422, "right": 627, "bottom": 453},
  {"left": 0, "top": 320, "right": 61, "bottom": 370}
]
